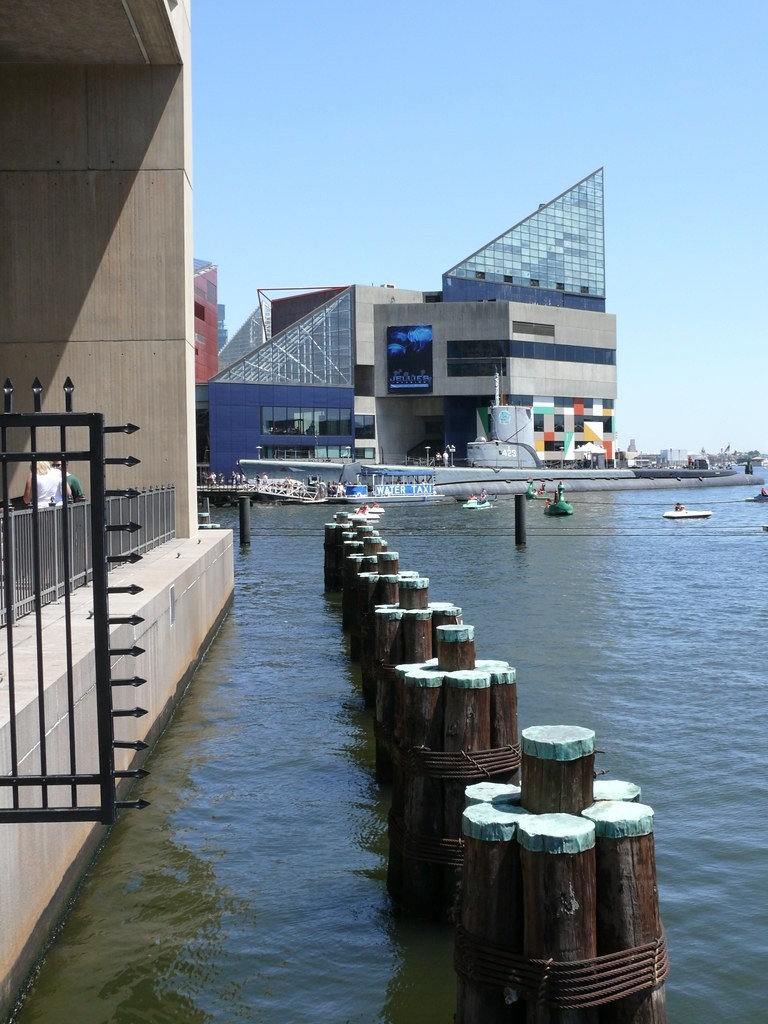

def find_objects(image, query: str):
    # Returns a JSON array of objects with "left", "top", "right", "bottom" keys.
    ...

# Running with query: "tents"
[{"left": 573, "top": 442, "right": 609, "bottom": 470}]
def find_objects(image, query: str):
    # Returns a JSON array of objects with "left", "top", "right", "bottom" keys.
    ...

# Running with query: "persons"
[
  {"left": 680, "top": 505, "right": 686, "bottom": 512},
  {"left": 356, "top": 506, "right": 366, "bottom": 515},
  {"left": 23, "top": 461, "right": 71, "bottom": 510},
  {"left": 761, "top": 487, "right": 768, "bottom": 497},
  {"left": 364, "top": 501, "right": 369, "bottom": 510},
  {"left": 675, "top": 502, "right": 681, "bottom": 512},
  {"left": 51, "top": 459, "right": 85, "bottom": 505},
  {"left": 436, "top": 452, "right": 443, "bottom": 467},
  {"left": 261, "top": 418, "right": 316, "bottom": 437},
  {"left": 371, "top": 500, "right": 379, "bottom": 508},
  {"left": 442, "top": 450, "right": 450, "bottom": 469},
  {"left": 208, "top": 469, "right": 432, "bottom": 503},
  {"left": 547, "top": 453, "right": 596, "bottom": 469},
  {"left": 466, "top": 485, "right": 560, "bottom": 511}
]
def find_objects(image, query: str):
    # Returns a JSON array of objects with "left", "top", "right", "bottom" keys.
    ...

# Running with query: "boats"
[
  {"left": 324, "top": 465, "right": 442, "bottom": 504},
  {"left": 462, "top": 496, "right": 495, "bottom": 510},
  {"left": 525, "top": 478, "right": 549, "bottom": 498},
  {"left": 333, "top": 512, "right": 381, "bottom": 523},
  {"left": 351, "top": 503, "right": 386, "bottom": 514},
  {"left": 663, "top": 509, "right": 714, "bottom": 519},
  {"left": 544, "top": 481, "right": 574, "bottom": 514},
  {"left": 745, "top": 493, "right": 767, "bottom": 503}
]
